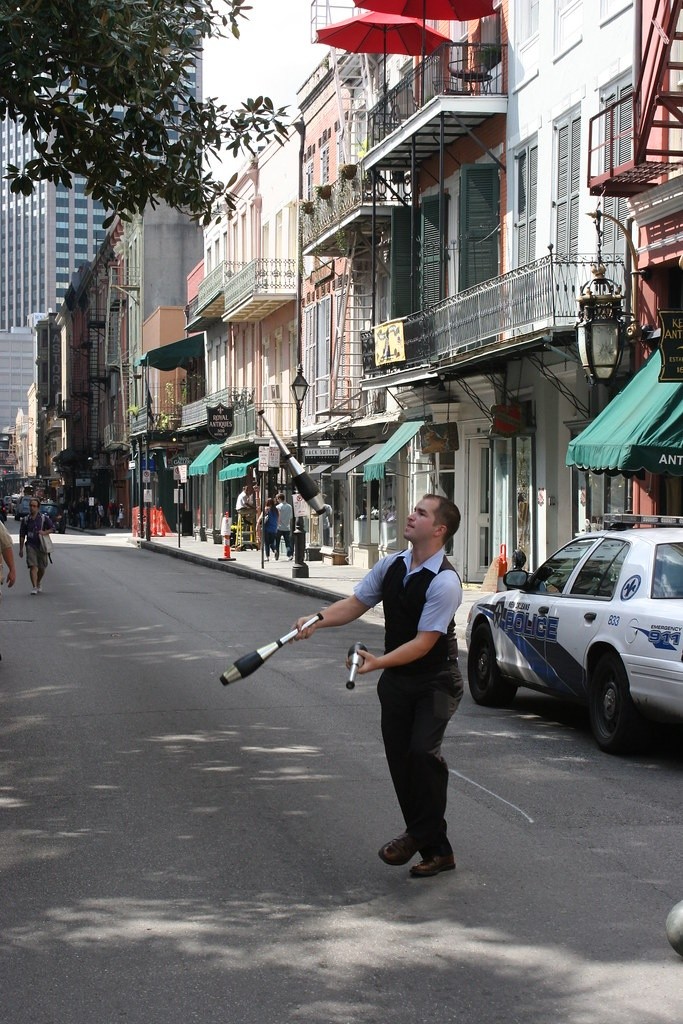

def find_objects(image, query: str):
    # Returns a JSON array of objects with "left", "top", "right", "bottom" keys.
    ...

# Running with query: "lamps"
[{"left": 579, "top": 205, "right": 654, "bottom": 385}]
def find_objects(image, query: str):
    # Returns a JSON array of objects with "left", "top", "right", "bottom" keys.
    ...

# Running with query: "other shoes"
[
  {"left": 31, "top": 586, "right": 42, "bottom": 594},
  {"left": 264, "top": 557, "right": 269, "bottom": 562},
  {"left": 286, "top": 555, "right": 294, "bottom": 562},
  {"left": 273, "top": 550, "right": 279, "bottom": 561},
  {"left": 244, "top": 519, "right": 253, "bottom": 525}
]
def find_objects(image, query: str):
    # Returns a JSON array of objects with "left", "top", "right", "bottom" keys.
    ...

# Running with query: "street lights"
[{"left": 291, "top": 367, "right": 312, "bottom": 578}]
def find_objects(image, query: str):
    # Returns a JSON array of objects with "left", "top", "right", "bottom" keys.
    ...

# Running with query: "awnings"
[
  {"left": 188, "top": 420, "right": 427, "bottom": 481},
  {"left": 134, "top": 332, "right": 204, "bottom": 371},
  {"left": 566, "top": 347, "right": 683, "bottom": 478}
]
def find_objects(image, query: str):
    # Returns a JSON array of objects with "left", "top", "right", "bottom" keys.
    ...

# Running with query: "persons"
[
  {"left": 235, "top": 486, "right": 258, "bottom": 525},
  {"left": 0, "top": 521, "right": 17, "bottom": 588},
  {"left": 290, "top": 495, "right": 465, "bottom": 878},
  {"left": 256, "top": 493, "right": 292, "bottom": 562},
  {"left": 11, "top": 492, "right": 127, "bottom": 530},
  {"left": 19, "top": 499, "right": 56, "bottom": 595}
]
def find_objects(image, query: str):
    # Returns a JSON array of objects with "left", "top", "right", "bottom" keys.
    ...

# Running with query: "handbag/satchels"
[{"left": 39, "top": 514, "right": 55, "bottom": 555}]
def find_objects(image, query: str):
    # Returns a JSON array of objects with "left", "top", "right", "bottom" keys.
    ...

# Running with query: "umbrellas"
[{"left": 317, "top": 0, "right": 495, "bottom": 137}]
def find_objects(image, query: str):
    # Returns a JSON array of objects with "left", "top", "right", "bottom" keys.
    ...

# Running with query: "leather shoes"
[
  {"left": 377, "top": 829, "right": 419, "bottom": 866},
  {"left": 408, "top": 853, "right": 456, "bottom": 876}
]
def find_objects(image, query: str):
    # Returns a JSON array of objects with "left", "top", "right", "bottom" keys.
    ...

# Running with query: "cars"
[
  {"left": 0, "top": 494, "right": 67, "bottom": 535},
  {"left": 464, "top": 513, "right": 683, "bottom": 758}
]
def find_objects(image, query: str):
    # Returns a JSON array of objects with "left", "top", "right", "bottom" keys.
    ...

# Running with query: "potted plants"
[
  {"left": 315, "top": 183, "right": 331, "bottom": 264},
  {"left": 336, "top": 164, "right": 358, "bottom": 256},
  {"left": 166, "top": 383, "right": 181, "bottom": 431},
  {"left": 297, "top": 199, "right": 314, "bottom": 278}
]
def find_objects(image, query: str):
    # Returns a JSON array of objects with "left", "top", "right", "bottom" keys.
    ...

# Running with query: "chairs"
[
  {"left": 432, "top": 73, "right": 459, "bottom": 96},
  {"left": 378, "top": 104, "right": 402, "bottom": 142},
  {"left": 450, "top": 45, "right": 500, "bottom": 93}
]
